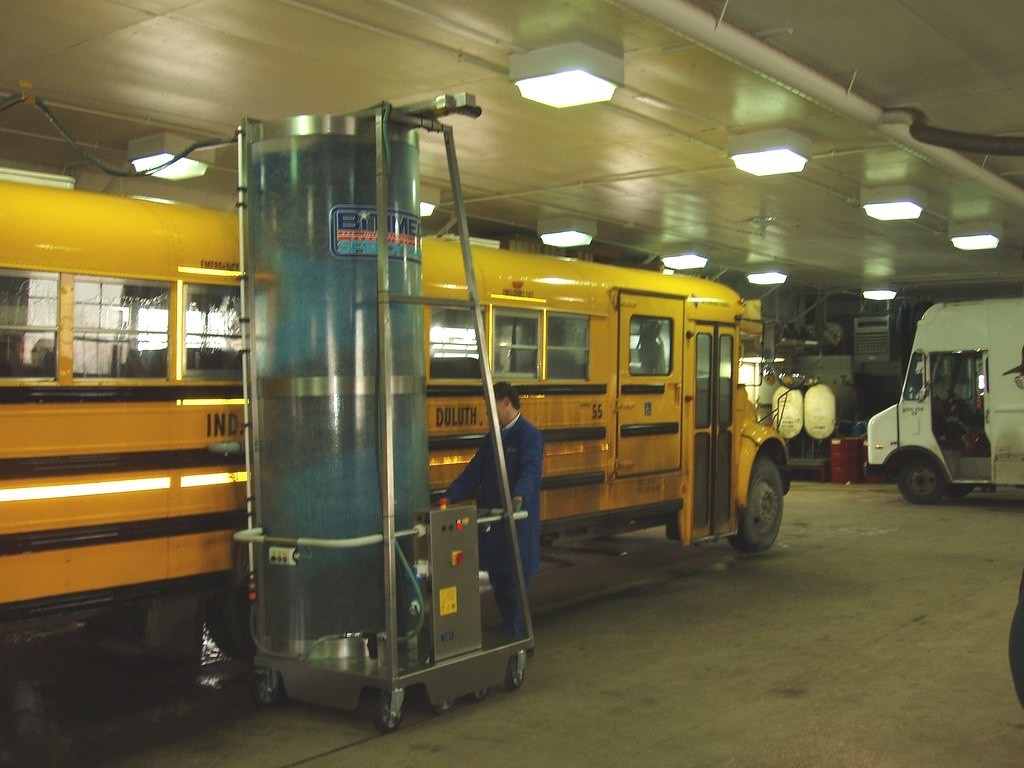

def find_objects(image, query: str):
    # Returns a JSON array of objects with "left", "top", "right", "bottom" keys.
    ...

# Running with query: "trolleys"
[{"left": 234, "top": 90, "right": 538, "bottom": 729}]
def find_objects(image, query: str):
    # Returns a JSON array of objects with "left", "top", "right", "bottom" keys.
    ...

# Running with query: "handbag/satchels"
[{"left": 961, "top": 432, "right": 990, "bottom": 457}]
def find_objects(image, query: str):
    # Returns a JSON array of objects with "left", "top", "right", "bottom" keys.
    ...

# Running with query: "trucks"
[{"left": 862, "top": 298, "right": 1024, "bottom": 504}]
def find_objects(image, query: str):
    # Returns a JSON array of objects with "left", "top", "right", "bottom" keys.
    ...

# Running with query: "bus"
[{"left": 1, "top": 181, "right": 808, "bottom": 664}]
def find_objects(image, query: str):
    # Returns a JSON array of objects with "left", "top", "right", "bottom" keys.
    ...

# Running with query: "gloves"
[{"left": 512, "top": 499, "right": 523, "bottom": 512}]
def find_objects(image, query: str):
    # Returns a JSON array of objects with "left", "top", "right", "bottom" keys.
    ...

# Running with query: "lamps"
[
  {"left": 418, "top": 183, "right": 444, "bottom": 220},
  {"left": 659, "top": 240, "right": 714, "bottom": 271},
  {"left": 726, "top": 127, "right": 812, "bottom": 178},
  {"left": 747, "top": 263, "right": 792, "bottom": 286},
  {"left": 858, "top": 281, "right": 902, "bottom": 301},
  {"left": 535, "top": 217, "right": 602, "bottom": 248},
  {"left": 508, "top": 42, "right": 626, "bottom": 111},
  {"left": 123, "top": 132, "right": 215, "bottom": 184},
  {"left": 946, "top": 219, "right": 1006, "bottom": 251},
  {"left": 857, "top": 185, "right": 928, "bottom": 223}
]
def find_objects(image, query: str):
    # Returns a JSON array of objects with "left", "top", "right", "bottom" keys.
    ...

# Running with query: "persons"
[{"left": 433, "top": 381, "right": 543, "bottom": 657}]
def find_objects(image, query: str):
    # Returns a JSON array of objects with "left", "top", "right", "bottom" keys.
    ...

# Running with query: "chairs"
[{"left": 33, "top": 338, "right": 232, "bottom": 374}]
